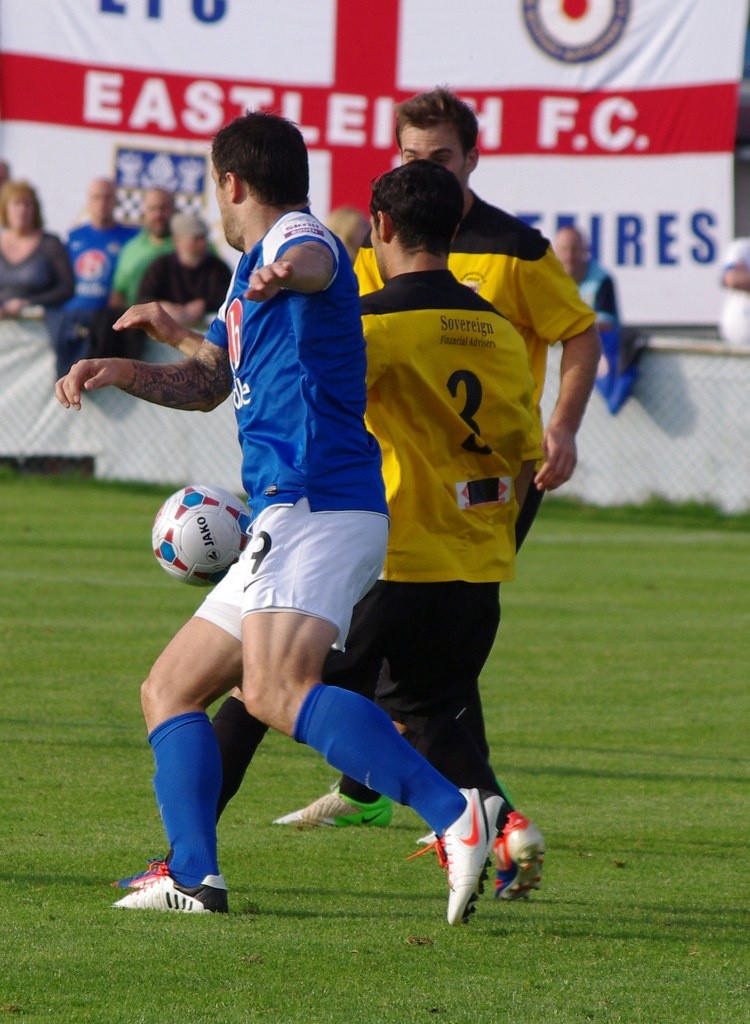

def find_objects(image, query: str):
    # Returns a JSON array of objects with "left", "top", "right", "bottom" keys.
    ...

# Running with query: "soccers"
[{"left": 149, "top": 484, "right": 254, "bottom": 587}]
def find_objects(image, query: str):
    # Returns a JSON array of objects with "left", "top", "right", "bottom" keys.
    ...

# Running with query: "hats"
[
  {"left": 169, "top": 210, "right": 207, "bottom": 239},
  {"left": 723, "top": 235, "right": 750, "bottom": 266}
]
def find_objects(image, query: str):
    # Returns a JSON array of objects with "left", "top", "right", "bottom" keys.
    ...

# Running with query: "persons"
[
  {"left": 272, "top": 89, "right": 602, "bottom": 827},
  {"left": 109, "top": 189, "right": 216, "bottom": 310},
  {"left": 0, "top": 182, "right": 75, "bottom": 319},
  {"left": 135, "top": 213, "right": 232, "bottom": 325},
  {"left": 64, "top": 179, "right": 142, "bottom": 310},
  {"left": 553, "top": 227, "right": 619, "bottom": 332},
  {"left": 55, "top": 112, "right": 510, "bottom": 929},
  {"left": 110, "top": 161, "right": 548, "bottom": 899},
  {"left": 721, "top": 236, "right": 750, "bottom": 344}
]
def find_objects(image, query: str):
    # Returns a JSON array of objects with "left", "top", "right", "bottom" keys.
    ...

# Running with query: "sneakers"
[
  {"left": 493, "top": 811, "right": 546, "bottom": 903},
  {"left": 110, "top": 859, "right": 170, "bottom": 890},
  {"left": 114, "top": 873, "right": 229, "bottom": 916},
  {"left": 436, "top": 788, "right": 509, "bottom": 926},
  {"left": 276, "top": 789, "right": 393, "bottom": 827}
]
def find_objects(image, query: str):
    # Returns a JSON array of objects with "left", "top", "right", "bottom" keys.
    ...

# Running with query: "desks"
[{"left": 0, "top": 313, "right": 750, "bottom": 519}]
